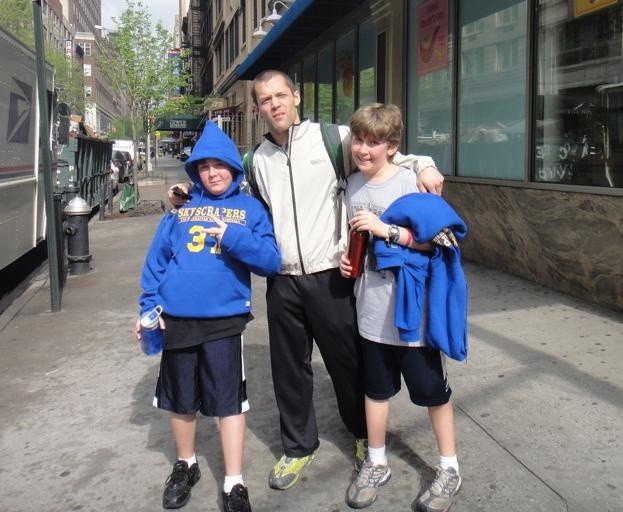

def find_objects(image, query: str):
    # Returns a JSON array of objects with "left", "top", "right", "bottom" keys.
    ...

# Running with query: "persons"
[
  {"left": 336, "top": 102, "right": 463, "bottom": 510},
  {"left": 114, "top": 152, "right": 140, "bottom": 213},
  {"left": 134, "top": 120, "right": 282, "bottom": 512},
  {"left": 165, "top": 69, "right": 445, "bottom": 492}
]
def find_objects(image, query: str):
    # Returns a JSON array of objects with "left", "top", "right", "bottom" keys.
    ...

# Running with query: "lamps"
[
  {"left": 250, "top": 15, "right": 278, "bottom": 40},
  {"left": 263, "top": 1, "right": 289, "bottom": 25}
]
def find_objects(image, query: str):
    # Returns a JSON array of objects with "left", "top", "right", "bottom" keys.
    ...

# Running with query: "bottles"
[
  {"left": 139, "top": 305, "right": 164, "bottom": 356},
  {"left": 346, "top": 226, "right": 370, "bottom": 280}
]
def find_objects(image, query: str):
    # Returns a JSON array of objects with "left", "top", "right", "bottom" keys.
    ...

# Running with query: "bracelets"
[{"left": 405, "top": 228, "right": 412, "bottom": 248}]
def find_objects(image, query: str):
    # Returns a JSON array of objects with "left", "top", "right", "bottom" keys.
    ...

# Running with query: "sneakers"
[
  {"left": 269, "top": 450, "right": 316, "bottom": 490},
  {"left": 354, "top": 439, "right": 368, "bottom": 471},
  {"left": 347, "top": 456, "right": 391, "bottom": 509},
  {"left": 415, "top": 464, "right": 462, "bottom": 511},
  {"left": 222, "top": 484, "right": 251, "bottom": 512},
  {"left": 163, "top": 461, "right": 200, "bottom": 509}
]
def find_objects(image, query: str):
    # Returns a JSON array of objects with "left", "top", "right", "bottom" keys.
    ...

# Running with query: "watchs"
[{"left": 388, "top": 224, "right": 400, "bottom": 244}]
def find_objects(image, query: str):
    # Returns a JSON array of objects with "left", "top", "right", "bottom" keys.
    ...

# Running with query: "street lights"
[{"left": 93, "top": 22, "right": 127, "bottom": 119}]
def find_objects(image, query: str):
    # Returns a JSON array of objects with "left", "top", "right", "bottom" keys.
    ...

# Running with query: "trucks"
[
  {"left": 54, "top": 112, "right": 115, "bottom": 215},
  {"left": 426, "top": 132, "right": 606, "bottom": 186},
  {"left": 0, "top": 27, "right": 71, "bottom": 276}
]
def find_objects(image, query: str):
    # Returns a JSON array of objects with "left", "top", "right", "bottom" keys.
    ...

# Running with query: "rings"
[
  {"left": 210, "top": 228, "right": 216, "bottom": 234},
  {"left": 217, "top": 235, "right": 221, "bottom": 240}
]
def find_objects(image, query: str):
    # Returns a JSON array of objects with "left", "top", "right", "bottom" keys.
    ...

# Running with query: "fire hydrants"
[{"left": 61, "top": 197, "right": 92, "bottom": 277}]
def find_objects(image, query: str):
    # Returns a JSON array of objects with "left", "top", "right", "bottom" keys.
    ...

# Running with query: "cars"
[{"left": 109, "top": 138, "right": 155, "bottom": 194}]
